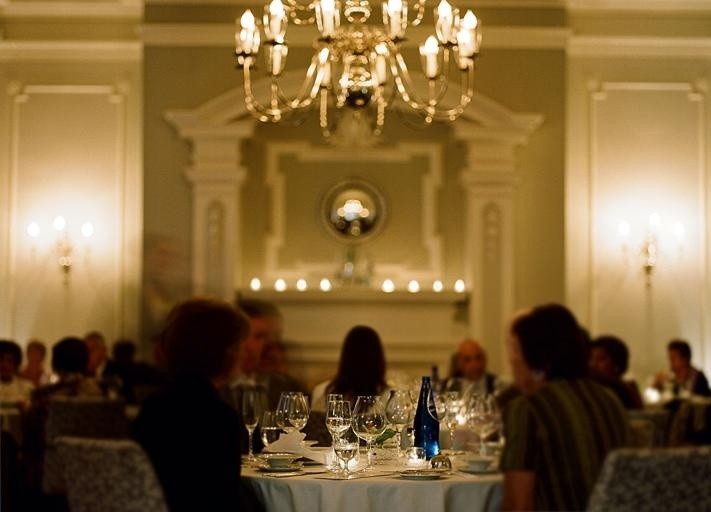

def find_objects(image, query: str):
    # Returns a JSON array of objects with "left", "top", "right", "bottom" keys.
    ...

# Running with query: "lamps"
[{"left": 232, "top": 0, "right": 484, "bottom": 136}]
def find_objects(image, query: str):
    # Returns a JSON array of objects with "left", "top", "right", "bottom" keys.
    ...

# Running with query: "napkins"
[{"left": 261, "top": 430, "right": 332, "bottom": 466}]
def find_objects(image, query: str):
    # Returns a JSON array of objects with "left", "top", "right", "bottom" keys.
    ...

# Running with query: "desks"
[
  {"left": 440, "top": 426, "right": 483, "bottom": 450},
  {"left": 240, "top": 444, "right": 504, "bottom": 510}
]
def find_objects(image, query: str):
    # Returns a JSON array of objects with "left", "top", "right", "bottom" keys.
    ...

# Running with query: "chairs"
[
  {"left": 587, "top": 392, "right": 711, "bottom": 510},
  {"left": 1, "top": 392, "right": 168, "bottom": 510}
]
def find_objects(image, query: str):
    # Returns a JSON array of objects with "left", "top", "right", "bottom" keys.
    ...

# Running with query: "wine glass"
[{"left": 240, "top": 372, "right": 507, "bottom": 479}]
